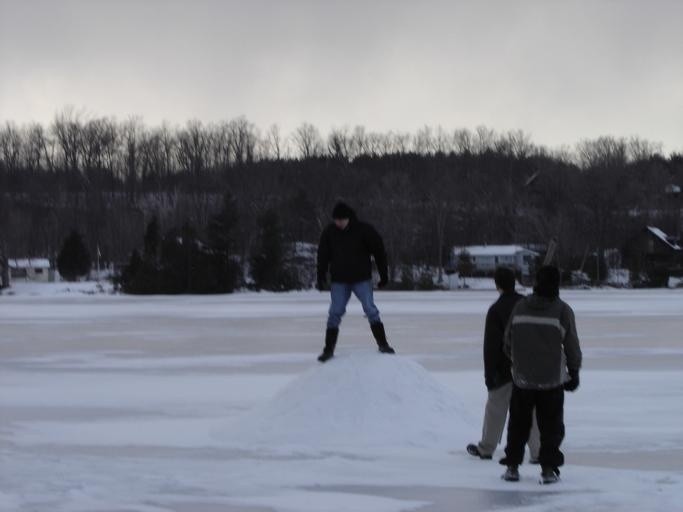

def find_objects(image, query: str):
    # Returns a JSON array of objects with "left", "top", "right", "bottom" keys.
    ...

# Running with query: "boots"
[
  {"left": 318, "top": 328, "right": 338, "bottom": 361},
  {"left": 371, "top": 323, "right": 395, "bottom": 353}
]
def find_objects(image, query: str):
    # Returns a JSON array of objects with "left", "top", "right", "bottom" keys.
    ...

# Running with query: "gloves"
[
  {"left": 564, "top": 372, "right": 579, "bottom": 391},
  {"left": 380, "top": 271, "right": 387, "bottom": 288},
  {"left": 316, "top": 272, "right": 327, "bottom": 291}
]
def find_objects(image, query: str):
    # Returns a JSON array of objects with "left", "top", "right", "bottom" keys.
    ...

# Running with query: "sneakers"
[{"left": 466, "top": 443, "right": 560, "bottom": 485}]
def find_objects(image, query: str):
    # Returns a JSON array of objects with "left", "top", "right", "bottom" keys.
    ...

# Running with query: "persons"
[
  {"left": 500, "top": 256, "right": 583, "bottom": 486},
  {"left": 315, "top": 200, "right": 393, "bottom": 360},
  {"left": 464, "top": 263, "right": 541, "bottom": 465}
]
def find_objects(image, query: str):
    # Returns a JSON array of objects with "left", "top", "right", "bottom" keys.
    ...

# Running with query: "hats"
[
  {"left": 534, "top": 266, "right": 559, "bottom": 296},
  {"left": 333, "top": 202, "right": 349, "bottom": 219}
]
